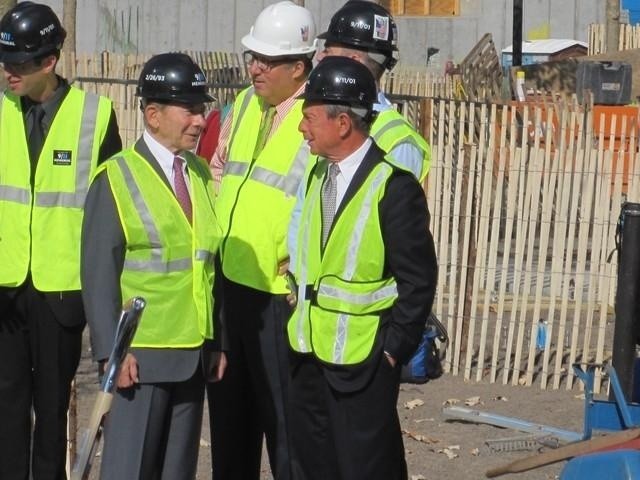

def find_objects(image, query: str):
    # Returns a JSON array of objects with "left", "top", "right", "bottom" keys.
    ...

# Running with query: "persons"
[
  {"left": 315, "top": 1, "right": 434, "bottom": 190},
  {"left": 202, "top": 0, "right": 318, "bottom": 479},
  {"left": 1, "top": 1, "right": 123, "bottom": 479},
  {"left": 78, "top": 52, "right": 234, "bottom": 479},
  {"left": 278, "top": 54, "right": 438, "bottom": 480}
]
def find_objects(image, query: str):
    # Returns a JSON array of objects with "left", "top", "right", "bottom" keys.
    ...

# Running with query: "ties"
[
  {"left": 320, "top": 164, "right": 338, "bottom": 250},
  {"left": 173, "top": 156, "right": 193, "bottom": 224},
  {"left": 251, "top": 103, "right": 277, "bottom": 165},
  {"left": 28, "top": 106, "right": 45, "bottom": 184}
]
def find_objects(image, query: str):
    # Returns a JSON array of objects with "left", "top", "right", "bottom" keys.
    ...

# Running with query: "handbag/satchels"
[{"left": 401, "top": 311, "right": 453, "bottom": 386}]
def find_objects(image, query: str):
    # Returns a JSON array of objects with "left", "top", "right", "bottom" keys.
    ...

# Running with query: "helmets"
[
  {"left": 0, "top": 1, "right": 69, "bottom": 64},
  {"left": 295, "top": 56, "right": 381, "bottom": 104},
  {"left": 136, "top": 52, "right": 218, "bottom": 105},
  {"left": 241, "top": 0, "right": 319, "bottom": 57},
  {"left": 315, "top": 0, "right": 400, "bottom": 53}
]
